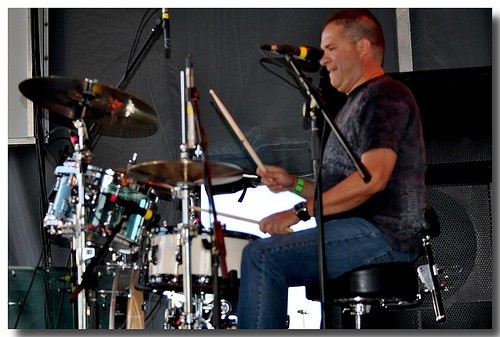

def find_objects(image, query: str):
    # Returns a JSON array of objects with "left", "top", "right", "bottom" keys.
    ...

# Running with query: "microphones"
[
  {"left": 102, "top": 192, "right": 161, "bottom": 224},
  {"left": 69, "top": 129, "right": 87, "bottom": 173},
  {"left": 162, "top": 8, "right": 170, "bottom": 50},
  {"left": 186, "top": 54, "right": 201, "bottom": 161},
  {"left": 261, "top": 44, "right": 321, "bottom": 60}
]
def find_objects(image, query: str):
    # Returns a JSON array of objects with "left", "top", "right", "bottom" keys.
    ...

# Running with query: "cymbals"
[
  {"left": 124, "top": 159, "right": 245, "bottom": 190},
  {"left": 19, "top": 77, "right": 160, "bottom": 138}
]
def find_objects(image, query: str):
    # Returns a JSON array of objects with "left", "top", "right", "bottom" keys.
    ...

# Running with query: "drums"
[
  {"left": 43, "top": 161, "right": 159, "bottom": 254},
  {"left": 148, "top": 226, "right": 263, "bottom": 295}
]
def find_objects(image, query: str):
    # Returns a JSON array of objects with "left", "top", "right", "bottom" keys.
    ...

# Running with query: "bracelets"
[
  {"left": 293, "top": 202, "right": 311, "bottom": 222},
  {"left": 293, "top": 178, "right": 305, "bottom": 194}
]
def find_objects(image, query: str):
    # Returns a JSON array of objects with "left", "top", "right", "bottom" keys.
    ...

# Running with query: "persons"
[{"left": 237, "top": 9, "right": 426, "bottom": 329}]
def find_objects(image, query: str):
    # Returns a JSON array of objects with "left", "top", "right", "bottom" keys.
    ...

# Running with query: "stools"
[{"left": 306, "top": 261, "right": 418, "bottom": 329}]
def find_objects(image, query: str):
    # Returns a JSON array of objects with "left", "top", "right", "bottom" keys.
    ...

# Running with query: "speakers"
[
  {"left": 384, "top": 67, "right": 493, "bottom": 164},
  {"left": 324, "top": 161, "right": 494, "bottom": 332}
]
